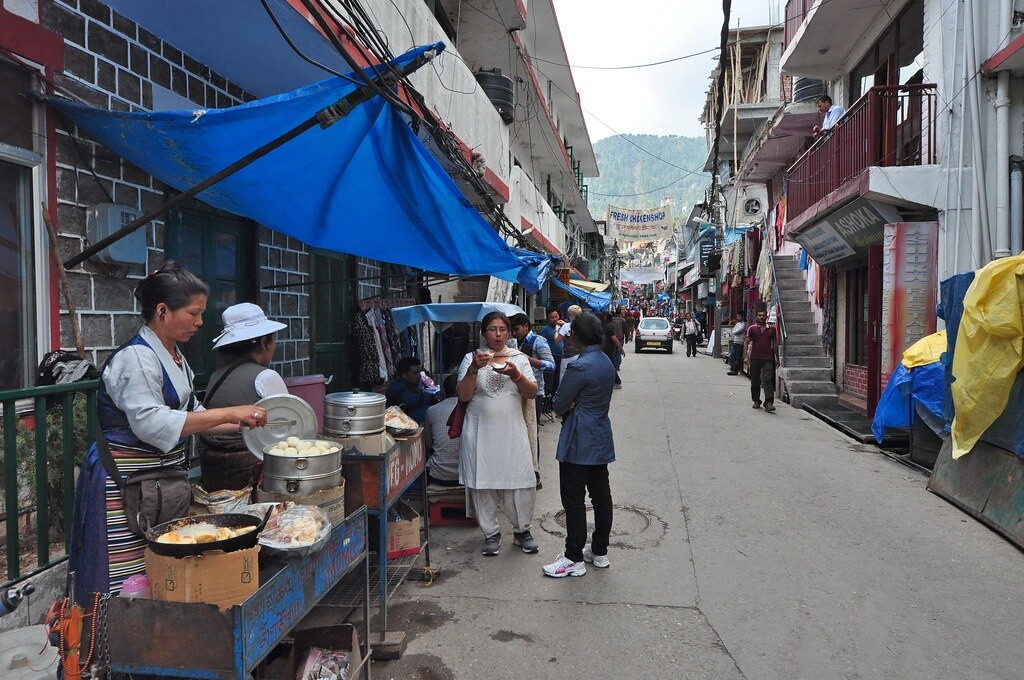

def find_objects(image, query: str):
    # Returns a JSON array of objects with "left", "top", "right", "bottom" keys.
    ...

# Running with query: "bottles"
[{"left": 119, "top": 575, "right": 151, "bottom": 599}]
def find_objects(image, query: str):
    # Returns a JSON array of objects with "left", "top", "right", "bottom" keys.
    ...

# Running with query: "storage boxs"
[
  {"left": 284, "top": 623, "right": 362, "bottom": 680},
  {"left": 372, "top": 497, "right": 421, "bottom": 559}
]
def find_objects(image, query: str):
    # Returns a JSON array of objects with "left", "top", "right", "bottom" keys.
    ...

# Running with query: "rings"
[{"left": 253, "top": 412, "right": 259, "bottom": 419}]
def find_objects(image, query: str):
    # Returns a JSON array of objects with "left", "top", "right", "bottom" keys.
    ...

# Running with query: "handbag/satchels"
[
  {"left": 696, "top": 334, "right": 703, "bottom": 343},
  {"left": 121, "top": 465, "right": 191, "bottom": 535},
  {"left": 446, "top": 401, "right": 467, "bottom": 439}
]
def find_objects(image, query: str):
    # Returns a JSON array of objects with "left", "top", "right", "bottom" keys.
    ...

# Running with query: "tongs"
[{"left": 238, "top": 420, "right": 297, "bottom": 427}]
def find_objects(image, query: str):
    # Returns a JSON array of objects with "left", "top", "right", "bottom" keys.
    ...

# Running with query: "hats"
[{"left": 212, "top": 303, "right": 287, "bottom": 351}]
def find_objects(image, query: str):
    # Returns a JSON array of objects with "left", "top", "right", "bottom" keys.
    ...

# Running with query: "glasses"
[{"left": 486, "top": 326, "right": 508, "bottom": 333}]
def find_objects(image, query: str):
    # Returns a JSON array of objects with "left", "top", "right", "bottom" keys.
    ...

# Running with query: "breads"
[
  {"left": 485, "top": 351, "right": 495, "bottom": 358},
  {"left": 158, "top": 525, "right": 256, "bottom": 544},
  {"left": 385, "top": 412, "right": 417, "bottom": 429},
  {"left": 285, "top": 518, "right": 322, "bottom": 543}
]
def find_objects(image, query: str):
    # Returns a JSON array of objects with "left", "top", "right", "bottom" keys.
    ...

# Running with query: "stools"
[{"left": 429, "top": 501, "right": 476, "bottom": 525}]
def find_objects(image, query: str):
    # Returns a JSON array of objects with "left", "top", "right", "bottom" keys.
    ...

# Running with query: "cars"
[{"left": 635, "top": 317, "right": 673, "bottom": 354}]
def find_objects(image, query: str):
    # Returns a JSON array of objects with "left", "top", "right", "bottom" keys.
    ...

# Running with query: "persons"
[
  {"left": 419, "top": 374, "right": 462, "bottom": 516},
  {"left": 744, "top": 306, "right": 780, "bottom": 412},
  {"left": 454, "top": 310, "right": 555, "bottom": 556},
  {"left": 537, "top": 305, "right": 626, "bottom": 418},
  {"left": 680, "top": 307, "right": 708, "bottom": 359},
  {"left": 202, "top": 303, "right": 289, "bottom": 410},
  {"left": 811, "top": 94, "right": 846, "bottom": 137},
  {"left": 387, "top": 357, "right": 430, "bottom": 411},
  {"left": 542, "top": 314, "right": 616, "bottom": 581},
  {"left": 620, "top": 298, "right": 676, "bottom": 345},
  {"left": 727, "top": 310, "right": 746, "bottom": 375},
  {"left": 67, "top": 261, "right": 267, "bottom": 608}
]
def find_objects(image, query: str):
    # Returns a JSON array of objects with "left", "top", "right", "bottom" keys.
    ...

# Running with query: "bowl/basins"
[{"left": 385, "top": 425, "right": 415, "bottom": 435}]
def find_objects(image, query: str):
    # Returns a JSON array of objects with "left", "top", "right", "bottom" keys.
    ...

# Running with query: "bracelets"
[
  {"left": 468, "top": 366, "right": 477, "bottom": 376},
  {"left": 511, "top": 372, "right": 521, "bottom": 382},
  {"left": 528, "top": 356, "right": 531, "bottom": 361},
  {"left": 554, "top": 330, "right": 559, "bottom": 334}
]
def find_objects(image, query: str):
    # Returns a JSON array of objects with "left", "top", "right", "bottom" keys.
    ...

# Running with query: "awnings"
[{"left": 39, "top": 41, "right": 558, "bottom": 290}]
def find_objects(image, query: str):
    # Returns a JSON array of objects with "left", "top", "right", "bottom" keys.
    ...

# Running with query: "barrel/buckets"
[{"left": 283, "top": 374, "right": 332, "bottom": 431}]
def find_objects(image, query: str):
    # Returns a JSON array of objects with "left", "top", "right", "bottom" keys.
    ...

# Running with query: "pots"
[
  {"left": 137, "top": 504, "right": 274, "bottom": 559},
  {"left": 324, "top": 389, "right": 387, "bottom": 438},
  {"left": 261, "top": 440, "right": 343, "bottom": 495}
]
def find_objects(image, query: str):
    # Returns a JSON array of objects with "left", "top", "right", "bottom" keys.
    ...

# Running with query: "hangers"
[{"left": 362, "top": 300, "right": 392, "bottom": 310}]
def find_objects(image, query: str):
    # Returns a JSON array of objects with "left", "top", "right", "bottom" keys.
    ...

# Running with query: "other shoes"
[
  {"left": 692, "top": 353, "right": 695, "bottom": 357},
  {"left": 686, "top": 353, "right": 690, "bottom": 357},
  {"left": 765, "top": 403, "right": 776, "bottom": 412},
  {"left": 615, "top": 384, "right": 621, "bottom": 388},
  {"left": 727, "top": 371, "right": 737, "bottom": 375},
  {"left": 537, "top": 484, "right": 541, "bottom": 489},
  {"left": 752, "top": 400, "right": 762, "bottom": 408}
]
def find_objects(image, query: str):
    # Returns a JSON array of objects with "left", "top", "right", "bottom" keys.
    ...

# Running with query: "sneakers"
[
  {"left": 583, "top": 548, "right": 610, "bottom": 567},
  {"left": 483, "top": 533, "right": 502, "bottom": 555},
  {"left": 542, "top": 553, "right": 587, "bottom": 577},
  {"left": 513, "top": 531, "right": 539, "bottom": 552}
]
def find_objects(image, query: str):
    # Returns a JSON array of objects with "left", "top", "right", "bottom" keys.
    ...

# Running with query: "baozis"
[{"left": 268, "top": 436, "right": 340, "bottom": 457}]
